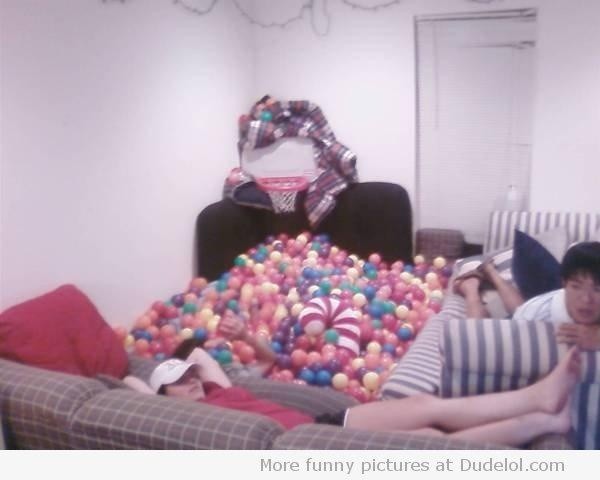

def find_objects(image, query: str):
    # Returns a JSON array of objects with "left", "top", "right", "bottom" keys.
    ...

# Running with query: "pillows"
[
  {"left": 453, "top": 244, "right": 514, "bottom": 288},
  {"left": 1, "top": 283, "right": 129, "bottom": 380},
  {"left": 511, "top": 226, "right": 562, "bottom": 299}
]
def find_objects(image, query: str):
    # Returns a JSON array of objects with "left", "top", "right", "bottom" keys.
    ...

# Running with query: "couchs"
[
  {"left": 0, "top": 350, "right": 576, "bottom": 449},
  {"left": 379, "top": 207, "right": 600, "bottom": 450}
]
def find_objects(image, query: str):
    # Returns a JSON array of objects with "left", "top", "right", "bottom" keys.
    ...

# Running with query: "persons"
[
  {"left": 453, "top": 239, "right": 600, "bottom": 350},
  {"left": 122, "top": 344, "right": 582, "bottom": 447},
  {"left": 114, "top": 229, "right": 453, "bottom": 404}
]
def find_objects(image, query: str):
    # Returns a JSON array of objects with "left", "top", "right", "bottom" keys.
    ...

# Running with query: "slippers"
[
  {"left": 453, "top": 270, "right": 485, "bottom": 294},
  {"left": 476, "top": 256, "right": 497, "bottom": 276}
]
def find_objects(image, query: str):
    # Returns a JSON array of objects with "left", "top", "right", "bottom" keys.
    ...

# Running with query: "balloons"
[{"left": 114, "top": 229, "right": 453, "bottom": 404}]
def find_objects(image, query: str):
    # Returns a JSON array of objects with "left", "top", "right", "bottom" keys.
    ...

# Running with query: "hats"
[{"left": 149, "top": 357, "right": 202, "bottom": 397}]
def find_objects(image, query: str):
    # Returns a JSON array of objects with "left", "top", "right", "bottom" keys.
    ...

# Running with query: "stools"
[{"left": 416, "top": 228, "right": 463, "bottom": 259}]
